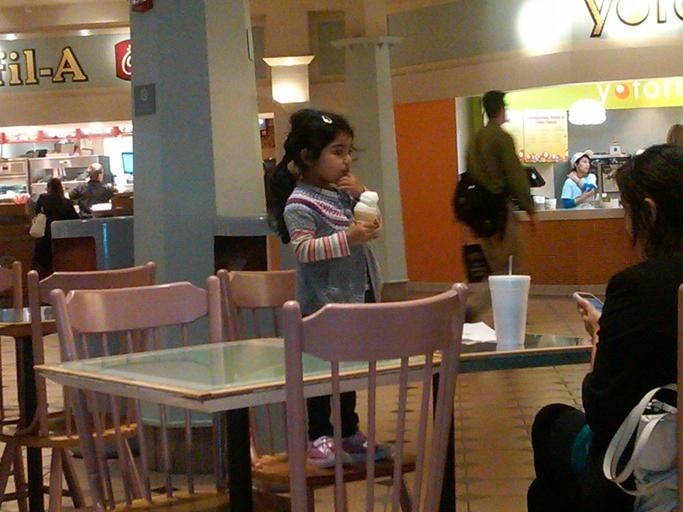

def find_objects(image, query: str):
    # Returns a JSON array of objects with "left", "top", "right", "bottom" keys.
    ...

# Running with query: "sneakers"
[{"left": 306, "top": 431, "right": 391, "bottom": 468}]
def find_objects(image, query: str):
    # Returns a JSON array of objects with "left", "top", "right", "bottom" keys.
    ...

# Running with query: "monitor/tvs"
[
  {"left": 525, "top": 166, "right": 546, "bottom": 188},
  {"left": 122, "top": 152, "right": 133, "bottom": 174}
]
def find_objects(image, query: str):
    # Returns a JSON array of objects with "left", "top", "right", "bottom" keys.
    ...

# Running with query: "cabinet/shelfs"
[
  {"left": 587, "top": 153, "right": 631, "bottom": 203},
  {"left": 0, "top": 155, "right": 110, "bottom": 203}
]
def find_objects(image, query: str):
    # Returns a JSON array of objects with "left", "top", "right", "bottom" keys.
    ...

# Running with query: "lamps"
[{"left": 263, "top": 55, "right": 315, "bottom": 103}]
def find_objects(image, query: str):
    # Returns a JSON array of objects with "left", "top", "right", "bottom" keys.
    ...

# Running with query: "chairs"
[
  {"left": 51, "top": 275, "right": 292, "bottom": 512},
  {"left": 0, "top": 261, "right": 73, "bottom": 511},
  {"left": 218, "top": 267, "right": 420, "bottom": 512},
  {"left": 251, "top": 283, "right": 468, "bottom": 512},
  {"left": 0, "top": 261, "right": 159, "bottom": 511}
]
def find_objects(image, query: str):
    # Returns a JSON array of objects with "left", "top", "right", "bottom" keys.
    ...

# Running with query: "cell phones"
[{"left": 573, "top": 291, "right": 604, "bottom": 313}]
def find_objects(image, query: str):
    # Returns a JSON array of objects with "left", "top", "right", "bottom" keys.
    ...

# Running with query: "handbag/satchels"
[
  {"left": 451, "top": 171, "right": 509, "bottom": 235},
  {"left": 29, "top": 206, "right": 47, "bottom": 238},
  {"left": 603, "top": 383, "right": 678, "bottom": 512}
]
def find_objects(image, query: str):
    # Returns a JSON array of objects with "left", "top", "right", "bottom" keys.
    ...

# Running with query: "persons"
[
  {"left": 526, "top": 143, "right": 683, "bottom": 512},
  {"left": 561, "top": 150, "right": 598, "bottom": 208},
  {"left": 466, "top": 90, "right": 538, "bottom": 323},
  {"left": 667, "top": 125, "right": 683, "bottom": 144},
  {"left": 267, "top": 108, "right": 391, "bottom": 469},
  {"left": 34, "top": 163, "right": 113, "bottom": 274}
]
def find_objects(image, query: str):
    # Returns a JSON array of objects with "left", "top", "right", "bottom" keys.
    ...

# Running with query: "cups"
[
  {"left": 610, "top": 145, "right": 622, "bottom": 155},
  {"left": 544, "top": 199, "right": 556, "bottom": 211},
  {"left": 488, "top": 274, "right": 531, "bottom": 348}
]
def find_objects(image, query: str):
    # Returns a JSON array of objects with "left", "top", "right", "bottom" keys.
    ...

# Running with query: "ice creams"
[{"left": 353, "top": 191, "right": 379, "bottom": 226}]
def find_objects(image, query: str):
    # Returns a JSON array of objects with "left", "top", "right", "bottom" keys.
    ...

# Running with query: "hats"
[{"left": 570, "top": 149, "right": 594, "bottom": 168}]
